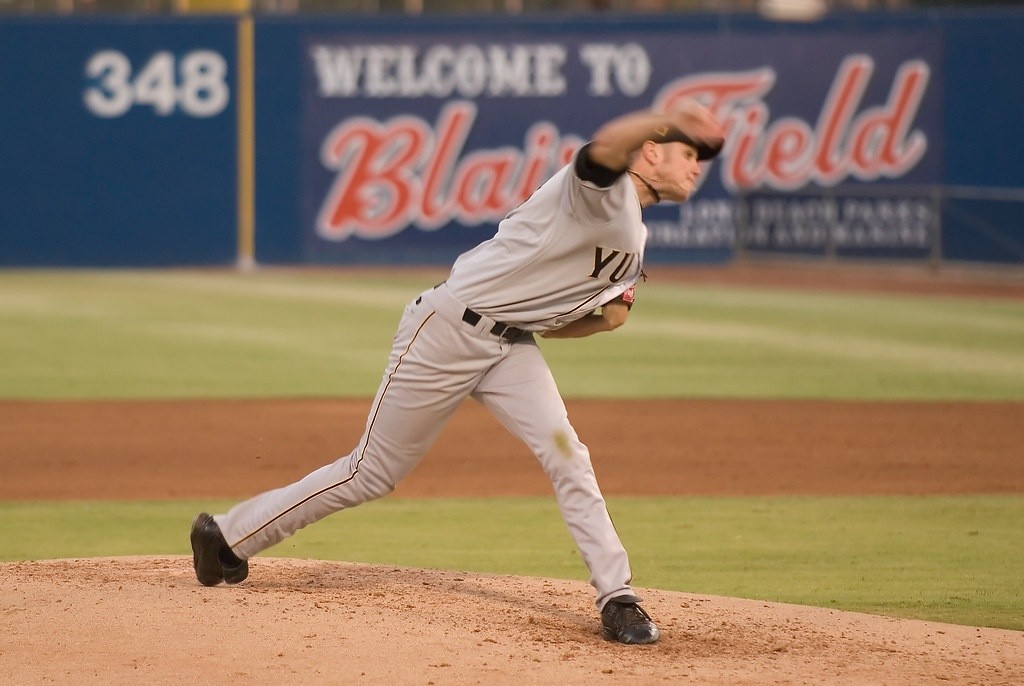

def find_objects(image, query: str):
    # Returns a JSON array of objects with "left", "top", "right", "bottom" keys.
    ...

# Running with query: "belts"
[{"left": 435, "top": 281, "right": 532, "bottom": 339}]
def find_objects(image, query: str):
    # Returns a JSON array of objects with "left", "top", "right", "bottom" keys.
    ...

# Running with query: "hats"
[{"left": 631, "top": 123, "right": 724, "bottom": 160}]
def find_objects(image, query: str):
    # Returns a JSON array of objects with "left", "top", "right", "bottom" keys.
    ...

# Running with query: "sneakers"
[
  {"left": 190, "top": 512, "right": 248, "bottom": 587},
  {"left": 600, "top": 595, "right": 660, "bottom": 644}
]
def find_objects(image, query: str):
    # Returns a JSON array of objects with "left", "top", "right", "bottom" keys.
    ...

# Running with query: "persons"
[{"left": 190, "top": 95, "right": 727, "bottom": 644}]
